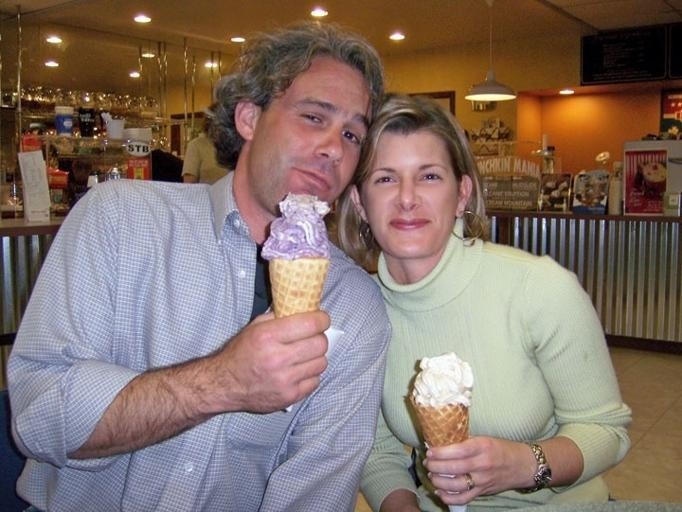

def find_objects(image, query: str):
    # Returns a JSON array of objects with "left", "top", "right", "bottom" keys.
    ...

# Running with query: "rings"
[{"left": 463, "top": 473, "right": 474, "bottom": 490}]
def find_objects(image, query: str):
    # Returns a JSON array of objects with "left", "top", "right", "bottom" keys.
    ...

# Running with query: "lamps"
[{"left": 465, "top": 0, "right": 517, "bottom": 101}]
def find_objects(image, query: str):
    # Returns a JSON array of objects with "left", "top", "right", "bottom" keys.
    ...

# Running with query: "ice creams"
[
  {"left": 261, "top": 191, "right": 331, "bottom": 319},
  {"left": 409, "top": 353, "right": 475, "bottom": 448}
]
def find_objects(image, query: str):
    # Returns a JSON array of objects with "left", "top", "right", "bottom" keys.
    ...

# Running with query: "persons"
[
  {"left": 324, "top": 96, "right": 632, "bottom": 512},
  {"left": 63, "top": 159, "right": 99, "bottom": 209},
  {"left": 181, "top": 102, "right": 230, "bottom": 185},
  {"left": 5, "top": 22, "right": 390, "bottom": 511}
]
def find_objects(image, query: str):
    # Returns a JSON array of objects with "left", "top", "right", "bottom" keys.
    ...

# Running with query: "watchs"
[{"left": 516, "top": 438, "right": 551, "bottom": 493}]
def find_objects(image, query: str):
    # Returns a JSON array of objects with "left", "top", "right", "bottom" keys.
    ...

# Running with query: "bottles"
[{"left": 606, "top": 176, "right": 623, "bottom": 216}]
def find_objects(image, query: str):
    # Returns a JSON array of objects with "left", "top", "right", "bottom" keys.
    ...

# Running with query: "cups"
[
  {"left": 78, "top": 106, "right": 96, "bottom": 138},
  {"left": 54, "top": 105, "right": 75, "bottom": 137},
  {"left": 105, "top": 118, "right": 125, "bottom": 141},
  {"left": 641, "top": 163, "right": 667, "bottom": 193}
]
